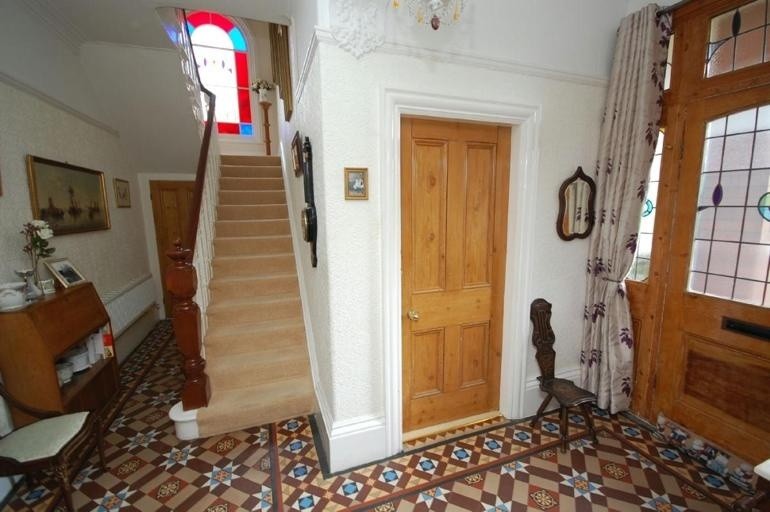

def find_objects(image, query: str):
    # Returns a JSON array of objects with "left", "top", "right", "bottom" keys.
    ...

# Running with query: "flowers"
[{"left": 250, "top": 78, "right": 275, "bottom": 90}]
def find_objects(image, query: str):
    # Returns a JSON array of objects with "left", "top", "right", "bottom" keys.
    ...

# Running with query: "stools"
[{"left": 0, "top": 384, "right": 105, "bottom": 512}]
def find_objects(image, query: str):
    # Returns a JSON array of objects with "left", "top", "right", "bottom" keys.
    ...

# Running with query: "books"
[
  {"left": 97, "top": 321, "right": 114, "bottom": 357},
  {"left": 92, "top": 331, "right": 106, "bottom": 360}
]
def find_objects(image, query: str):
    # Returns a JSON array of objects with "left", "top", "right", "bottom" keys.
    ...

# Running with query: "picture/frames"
[
  {"left": 290, "top": 130, "right": 303, "bottom": 175},
  {"left": 44, "top": 257, "right": 87, "bottom": 289},
  {"left": 25, "top": 154, "right": 112, "bottom": 236},
  {"left": 114, "top": 177, "right": 131, "bottom": 208},
  {"left": 344, "top": 167, "right": 369, "bottom": 200}
]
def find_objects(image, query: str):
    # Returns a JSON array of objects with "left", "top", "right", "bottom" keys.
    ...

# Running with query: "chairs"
[{"left": 529, "top": 299, "right": 600, "bottom": 454}]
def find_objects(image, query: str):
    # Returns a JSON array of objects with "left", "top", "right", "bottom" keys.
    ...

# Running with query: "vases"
[
  {"left": 259, "top": 89, "right": 269, "bottom": 102},
  {"left": 20, "top": 219, "right": 56, "bottom": 282}
]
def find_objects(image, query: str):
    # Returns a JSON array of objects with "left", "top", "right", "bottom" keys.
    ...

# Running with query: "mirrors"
[{"left": 557, "top": 165, "right": 597, "bottom": 241}]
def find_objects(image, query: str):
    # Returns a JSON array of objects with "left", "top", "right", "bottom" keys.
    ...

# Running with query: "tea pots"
[{"left": 38, "top": 279, "right": 56, "bottom": 295}]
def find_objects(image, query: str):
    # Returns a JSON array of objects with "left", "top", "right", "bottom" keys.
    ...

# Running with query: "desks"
[{"left": 0, "top": 280, "right": 117, "bottom": 435}]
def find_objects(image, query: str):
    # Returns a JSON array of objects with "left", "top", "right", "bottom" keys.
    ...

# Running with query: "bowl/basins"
[
  {"left": 0, "top": 282, "right": 29, "bottom": 311},
  {"left": 63, "top": 348, "right": 91, "bottom": 373},
  {"left": 54, "top": 362, "right": 73, "bottom": 384}
]
